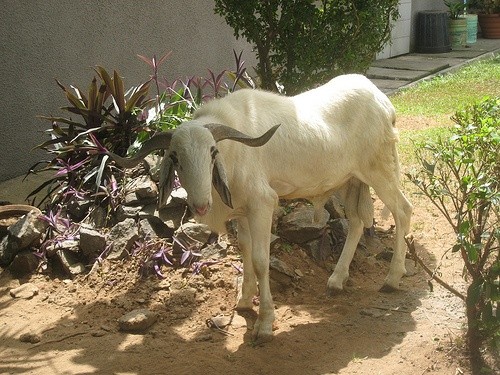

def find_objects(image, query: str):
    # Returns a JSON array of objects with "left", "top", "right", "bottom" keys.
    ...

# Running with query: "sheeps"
[{"left": 157, "top": 73, "right": 413, "bottom": 347}]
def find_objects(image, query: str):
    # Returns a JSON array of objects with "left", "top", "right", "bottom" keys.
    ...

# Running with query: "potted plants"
[{"left": 443, "top": 0, "right": 500, "bottom": 50}]
